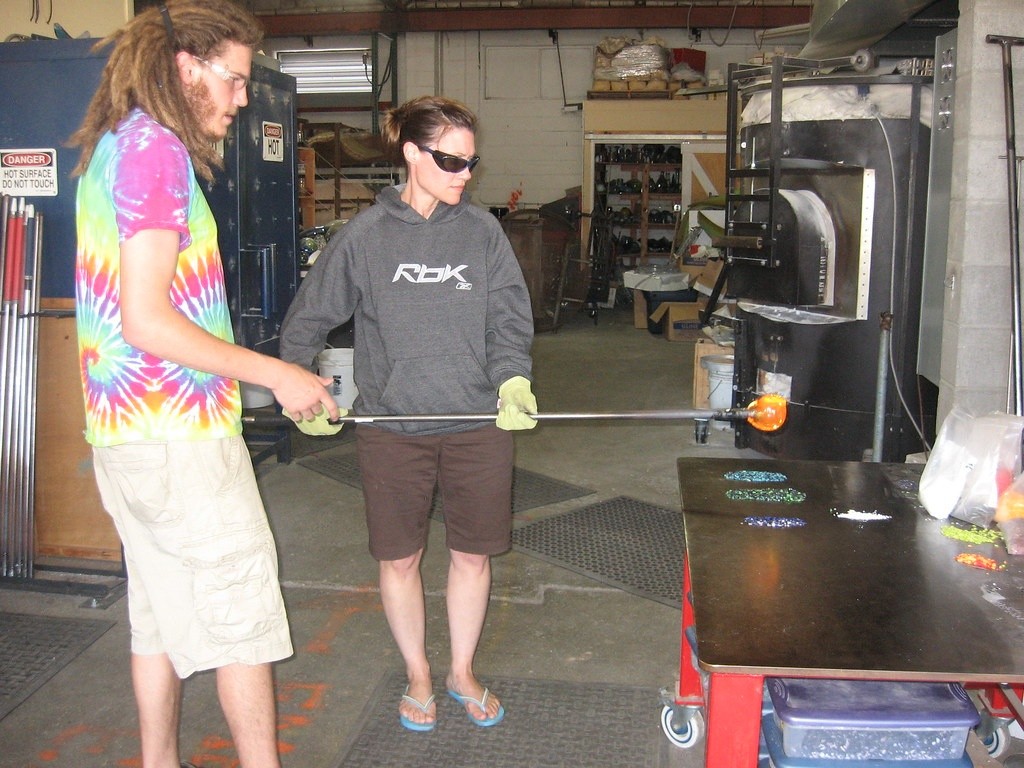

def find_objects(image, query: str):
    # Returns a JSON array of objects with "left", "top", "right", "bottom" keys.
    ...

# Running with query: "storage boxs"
[
  {"left": 694, "top": 337, "right": 734, "bottom": 412},
  {"left": 623, "top": 260, "right": 736, "bottom": 342},
  {"left": 684, "top": 591, "right": 981, "bottom": 768}
]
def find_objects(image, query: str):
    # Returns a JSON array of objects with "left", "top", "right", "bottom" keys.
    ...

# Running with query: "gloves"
[
  {"left": 282, "top": 405, "right": 348, "bottom": 436},
  {"left": 496, "top": 375, "right": 539, "bottom": 430}
]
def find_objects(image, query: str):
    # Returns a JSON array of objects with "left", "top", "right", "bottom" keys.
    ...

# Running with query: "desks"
[{"left": 676, "top": 458, "right": 1023, "bottom": 768}]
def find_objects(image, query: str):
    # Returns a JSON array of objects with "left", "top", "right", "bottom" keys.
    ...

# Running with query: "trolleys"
[{"left": 661, "top": 456, "right": 1024, "bottom": 768}]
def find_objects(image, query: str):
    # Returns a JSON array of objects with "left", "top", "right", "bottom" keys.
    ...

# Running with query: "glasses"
[
  {"left": 418, "top": 146, "right": 480, "bottom": 173},
  {"left": 190, "top": 54, "right": 252, "bottom": 90}
]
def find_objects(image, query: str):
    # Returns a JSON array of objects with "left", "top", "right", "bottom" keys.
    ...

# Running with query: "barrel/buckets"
[
  {"left": 239, "top": 381, "right": 275, "bottom": 409},
  {"left": 641, "top": 289, "right": 698, "bottom": 335},
  {"left": 317, "top": 347, "right": 360, "bottom": 409},
  {"left": 707, "top": 371, "right": 734, "bottom": 430}
]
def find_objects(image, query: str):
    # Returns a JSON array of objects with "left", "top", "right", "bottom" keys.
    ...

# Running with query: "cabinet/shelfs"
[{"left": 591, "top": 140, "right": 684, "bottom": 310}]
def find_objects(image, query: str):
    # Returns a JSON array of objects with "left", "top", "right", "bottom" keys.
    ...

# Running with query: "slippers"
[
  {"left": 401, "top": 683, "right": 436, "bottom": 732},
  {"left": 445, "top": 688, "right": 505, "bottom": 727}
]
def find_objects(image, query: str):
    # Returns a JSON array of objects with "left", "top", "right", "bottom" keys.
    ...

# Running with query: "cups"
[{"left": 488, "top": 206, "right": 509, "bottom": 222}]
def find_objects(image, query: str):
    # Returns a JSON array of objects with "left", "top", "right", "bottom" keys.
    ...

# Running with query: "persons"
[
  {"left": 61, "top": 0, "right": 341, "bottom": 768},
  {"left": 280, "top": 95, "right": 538, "bottom": 732}
]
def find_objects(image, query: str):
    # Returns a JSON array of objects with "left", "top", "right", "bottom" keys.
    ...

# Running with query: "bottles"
[{"left": 649, "top": 171, "right": 671, "bottom": 194}]
